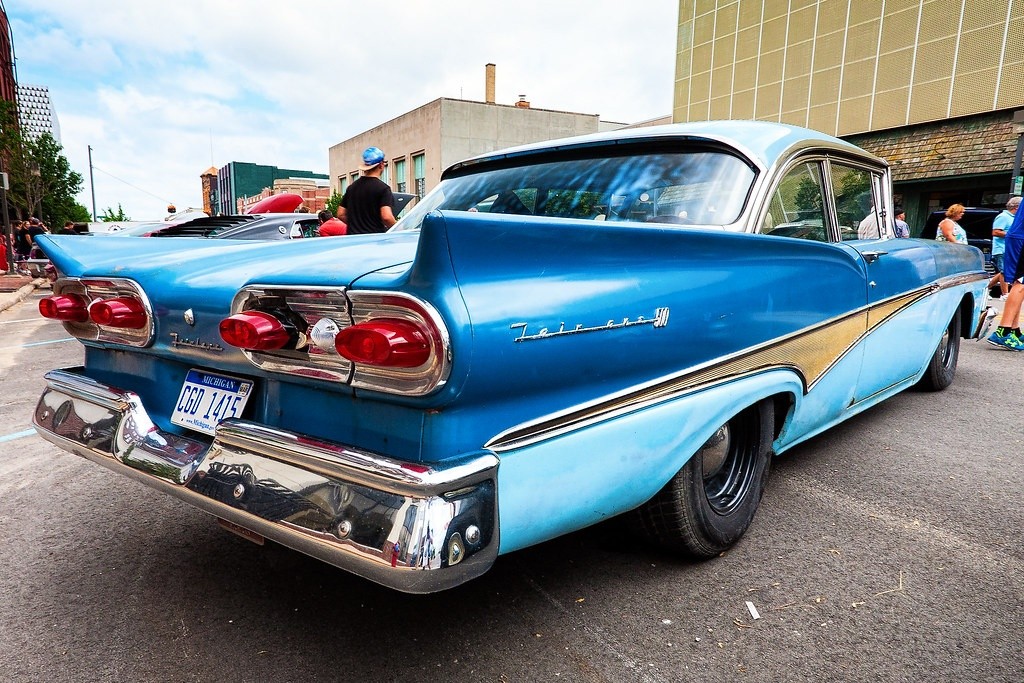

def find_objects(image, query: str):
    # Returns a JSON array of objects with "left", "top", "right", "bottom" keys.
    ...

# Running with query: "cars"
[
  {"left": 26, "top": 242, "right": 57, "bottom": 279},
  {"left": 109, "top": 213, "right": 328, "bottom": 239},
  {"left": 33, "top": 117, "right": 986, "bottom": 597}
]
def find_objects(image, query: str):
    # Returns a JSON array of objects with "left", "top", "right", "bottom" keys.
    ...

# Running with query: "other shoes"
[
  {"left": 988, "top": 289, "right": 992, "bottom": 300},
  {"left": 19, "top": 269, "right": 31, "bottom": 275},
  {"left": 998, "top": 294, "right": 1009, "bottom": 301}
]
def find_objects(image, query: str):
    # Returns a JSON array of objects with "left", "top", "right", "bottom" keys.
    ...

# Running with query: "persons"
[
  {"left": 9, "top": 217, "right": 50, "bottom": 275},
  {"left": 337, "top": 147, "right": 399, "bottom": 235},
  {"left": 58, "top": 221, "right": 78, "bottom": 235},
  {"left": 935, "top": 204, "right": 967, "bottom": 245},
  {"left": 986, "top": 197, "right": 1024, "bottom": 301},
  {"left": 858, "top": 198, "right": 883, "bottom": 240},
  {"left": 299, "top": 206, "right": 311, "bottom": 214},
  {"left": 988, "top": 196, "right": 1024, "bottom": 354},
  {"left": 315, "top": 210, "right": 347, "bottom": 237},
  {"left": 894, "top": 207, "right": 911, "bottom": 238}
]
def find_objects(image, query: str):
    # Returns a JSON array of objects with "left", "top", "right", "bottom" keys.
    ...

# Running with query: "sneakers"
[{"left": 987, "top": 328, "right": 1024, "bottom": 351}]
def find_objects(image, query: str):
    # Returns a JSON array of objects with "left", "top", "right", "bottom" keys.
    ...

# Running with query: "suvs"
[{"left": 918, "top": 212, "right": 1001, "bottom": 284}]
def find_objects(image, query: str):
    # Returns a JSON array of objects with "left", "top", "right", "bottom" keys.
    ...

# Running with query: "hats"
[{"left": 359, "top": 147, "right": 384, "bottom": 171}]
